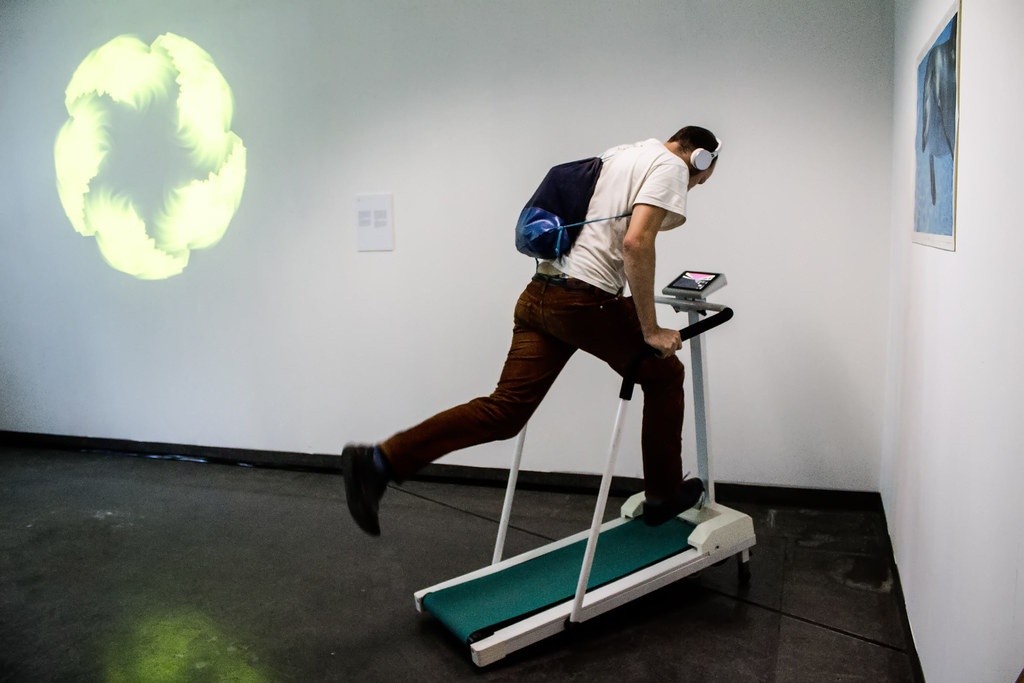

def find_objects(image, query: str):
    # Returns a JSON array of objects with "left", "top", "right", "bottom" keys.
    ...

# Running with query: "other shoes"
[
  {"left": 642, "top": 471, "right": 704, "bottom": 525},
  {"left": 342, "top": 444, "right": 388, "bottom": 536}
]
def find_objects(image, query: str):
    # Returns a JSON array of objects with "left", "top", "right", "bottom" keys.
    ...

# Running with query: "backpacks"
[{"left": 516, "top": 142, "right": 632, "bottom": 273}]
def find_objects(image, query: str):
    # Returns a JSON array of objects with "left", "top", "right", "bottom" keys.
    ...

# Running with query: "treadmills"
[{"left": 412, "top": 269, "right": 758, "bottom": 668}]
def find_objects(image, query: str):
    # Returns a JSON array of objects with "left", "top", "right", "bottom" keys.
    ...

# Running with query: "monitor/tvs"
[{"left": 662, "top": 271, "right": 728, "bottom": 299}]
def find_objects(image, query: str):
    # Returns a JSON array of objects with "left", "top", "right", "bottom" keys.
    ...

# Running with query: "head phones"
[{"left": 690, "top": 138, "right": 723, "bottom": 171}]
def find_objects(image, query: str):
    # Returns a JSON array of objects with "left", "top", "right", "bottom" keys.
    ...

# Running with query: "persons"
[{"left": 341, "top": 126, "right": 719, "bottom": 538}]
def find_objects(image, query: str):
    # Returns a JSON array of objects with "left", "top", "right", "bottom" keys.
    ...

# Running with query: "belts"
[{"left": 532, "top": 271, "right": 568, "bottom": 289}]
